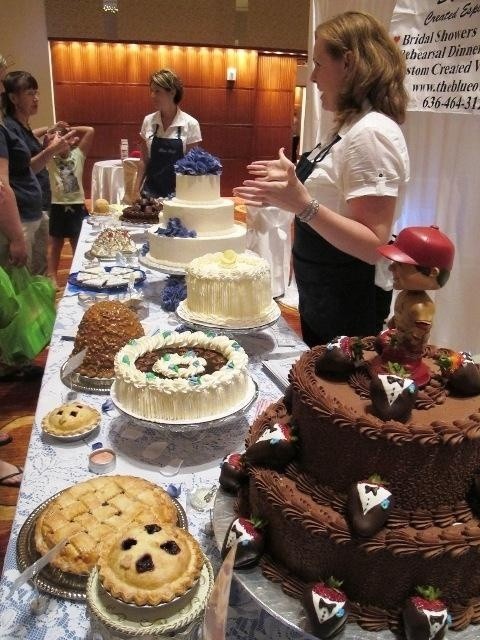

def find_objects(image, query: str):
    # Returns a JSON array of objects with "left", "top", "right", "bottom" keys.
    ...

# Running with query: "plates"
[
  {"left": 95, "top": 570, "right": 200, "bottom": 610},
  {"left": 86, "top": 542, "right": 214, "bottom": 637},
  {"left": 17, "top": 478, "right": 184, "bottom": 603},
  {"left": 41, "top": 410, "right": 101, "bottom": 441}
]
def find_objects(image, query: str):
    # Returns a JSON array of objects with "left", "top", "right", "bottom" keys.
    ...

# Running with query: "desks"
[
  {"left": 2, "top": 208, "right": 314, "bottom": 637},
  {"left": 90, "top": 158, "right": 143, "bottom": 204}
]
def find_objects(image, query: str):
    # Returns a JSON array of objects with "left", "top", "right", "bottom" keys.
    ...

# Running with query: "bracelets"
[{"left": 296, "top": 199, "right": 319, "bottom": 223}]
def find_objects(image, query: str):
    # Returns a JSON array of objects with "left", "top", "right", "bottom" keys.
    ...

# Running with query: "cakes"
[{"left": 32, "top": 144, "right": 480, "bottom": 640}]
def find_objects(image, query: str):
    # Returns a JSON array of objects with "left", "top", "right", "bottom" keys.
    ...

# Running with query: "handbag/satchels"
[{"left": 0, "top": 264, "right": 58, "bottom": 368}]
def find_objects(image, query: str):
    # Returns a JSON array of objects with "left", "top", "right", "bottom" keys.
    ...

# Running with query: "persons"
[
  {"left": 131, "top": 67, "right": 203, "bottom": 206},
  {"left": 2, "top": 71, "right": 77, "bottom": 277},
  {"left": 232, "top": 11, "right": 411, "bottom": 349},
  {"left": 31, "top": 120, "right": 95, "bottom": 292},
  {"left": 0, "top": 432, "right": 24, "bottom": 487},
  {"left": 0, "top": 55, "right": 42, "bottom": 268}
]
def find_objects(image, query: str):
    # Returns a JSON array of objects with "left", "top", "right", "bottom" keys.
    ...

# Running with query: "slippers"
[{"left": 0, "top": 460, "right": 26, "bottom": 488}]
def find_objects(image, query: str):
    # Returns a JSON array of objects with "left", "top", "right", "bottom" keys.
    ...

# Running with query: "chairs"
[
  {"left": 243, "top": 200, "right": 293, "bottom": 301},
  {"left": 118, "top": 158, "right": 147, "bottom": 205}
]
